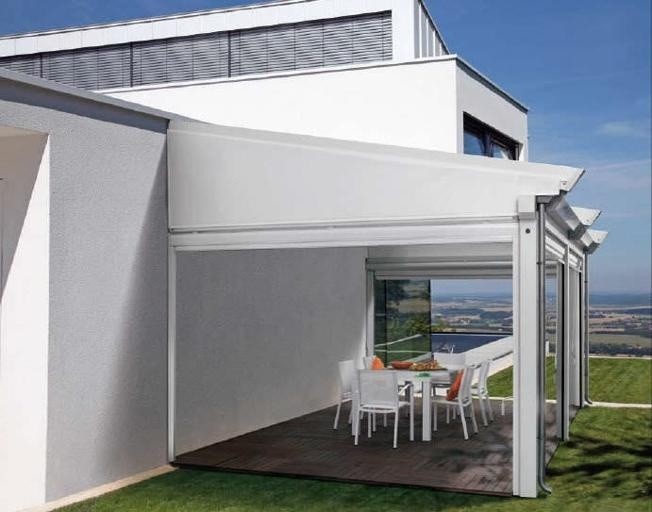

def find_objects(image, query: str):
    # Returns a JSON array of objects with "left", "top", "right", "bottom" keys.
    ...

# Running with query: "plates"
[{"left": 390, "top": 361, "right": 413, "bottom": 370}]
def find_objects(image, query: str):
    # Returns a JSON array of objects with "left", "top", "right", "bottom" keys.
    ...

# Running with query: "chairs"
[{"left": 330, "top": 354, "right": 495, "bottom": 449}]
